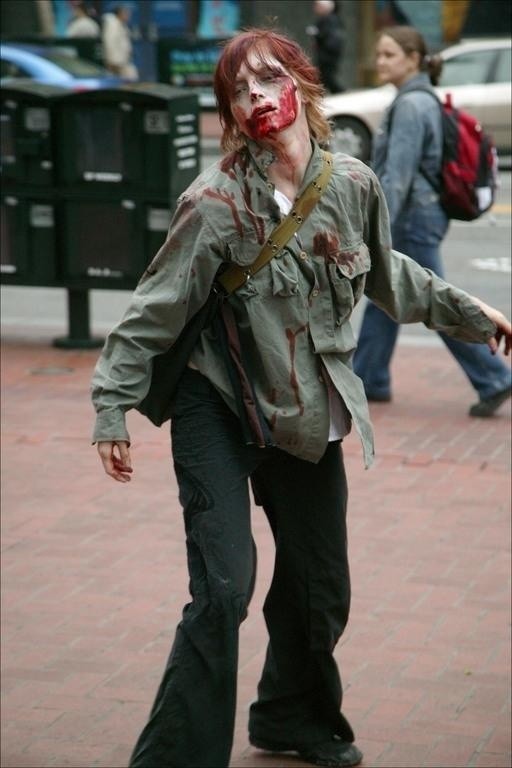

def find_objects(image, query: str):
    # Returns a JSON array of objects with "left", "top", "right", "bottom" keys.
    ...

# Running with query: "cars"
[
  {"left": 1, "top": 41, "right": 132, "bottom": 88},
  {"left": 303, "top": 34, "right": 512, "bottom": 167}
]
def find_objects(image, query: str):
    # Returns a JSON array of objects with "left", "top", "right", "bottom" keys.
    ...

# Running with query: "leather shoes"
[
  {"left": 357, "top": 375, "right": 392, "bottom": 402},
  {"left": 469, "top": 384, "right": 512, "bottom": 416}
]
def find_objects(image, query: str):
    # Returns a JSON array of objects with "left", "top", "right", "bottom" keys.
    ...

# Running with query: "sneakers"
[{"left": 246, "top": 719, "right": 361, "bottom": 767}]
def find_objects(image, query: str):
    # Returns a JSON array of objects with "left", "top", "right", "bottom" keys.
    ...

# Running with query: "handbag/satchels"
[{"left": 134, "top": 279, "right": 229, "bottom": 429}]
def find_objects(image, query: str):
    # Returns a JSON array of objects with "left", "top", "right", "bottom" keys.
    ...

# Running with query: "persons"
[
  {"left": 101, "top": 4, "right": 139, "bottom": 84},
  {"left": 350, "top": 24, "right": 512, "bottom": 417},
  {"left": 305, "top": 0, "right": 347, "bottom": 95},
  {"left": 88, "top": 28, "right": 512, "bottom": 768},
  {"left": 64, "top": 2, "right": 100, "bottom": 39}
]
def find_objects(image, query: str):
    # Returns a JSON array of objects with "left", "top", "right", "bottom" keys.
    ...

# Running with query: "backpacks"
[{"left": 389, "top": 88, "right": 500, "bottom": 224}]
